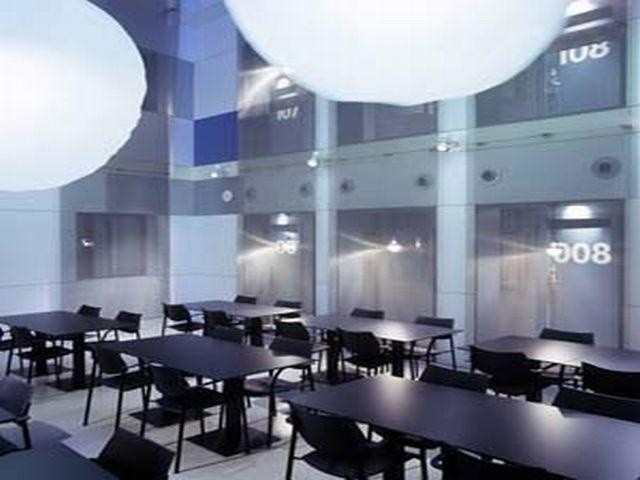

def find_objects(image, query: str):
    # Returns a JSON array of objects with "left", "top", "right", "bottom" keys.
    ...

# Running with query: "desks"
[
  {"left": 279, "top": 312, "right": 465, "bottom": 380},
  {"left": 182, "top": 300, "right": 304, "bottom": 349},
  {"left": 279, "top": 373, "right": 640, "bottom": 480},
  {"left": 99, "top": 334, "right": 316, "bottom": 458},
  {"left": 469, "top": 334, "right": 640, "bottom": 373},
  {"left": 0, "top": 310, "right": 132, "bottom": 392}
]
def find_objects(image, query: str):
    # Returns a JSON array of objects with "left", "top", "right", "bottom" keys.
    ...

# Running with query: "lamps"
[
  {"left": 435, "top": 136, "right": 457, "bottom": 152},
  {"left": 210, "top": 163, "right": 232, "bottom": 179},
  {"left": 307, "top": 150, "right": 322, "bottom": 169}
]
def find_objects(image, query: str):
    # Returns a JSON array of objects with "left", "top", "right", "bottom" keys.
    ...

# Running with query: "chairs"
[
  {"left": 539, "top": 328, "right": 595, "bottom": 373},
  {"left": 468, "top": 344, "right": 572, "bottom": 398},
  {"left": 160, "top": 322, "right": 252, "bottom": 409},
  {"left": 161, "top": 302, "right": 204, "bottom": 336},
  {"left": 85, "top": 428, "right": 177, "bottom": 480},
  {"left": 368, "top": 362, "right": 492, "bottom": 480},
  {"left": 385, "top": 314, "right": 459, "bottom": 378},
  {"left": 145, "top": 363, "right": 251, "bottom": 474},
  {"left": 4, "top": 325, "right": 73, "bottom": 386},
  {"left": 551, "top": 386, "right": 640, "bottom": 425},
  {"left": 272, "top": 317, "right": 331, "bottom": 391},
  {"left": 199, "top": 304, "right": 248, "bottom": 339},
  {"left": 317, "top": 307, "right": 392, "bottom": 374},
  {"left": 429, "top": 441, "right": 547, "bottom": 480},
  {"left": 233, "top": 293, "right": 257, "bottom": 305},
  {"left": 83, "top": 345, "right": 155, "bottom": 432},
  {"left": 0, "top": 374, "right": 36, "bottom": 451},
  {"left": 262, "top": 299, "right": 302, "bottom": 332},
  {"left": 0, "top": 325, "right": 34, "bottom": 375},
  {"left": 47, "top": 305, "right": 103, "bottom": 370},
  {"left": 580, "top": 361, "right": 640, "bottom": 400},
  {"left": 217, "top": 335, "right": 317, "bottom": 450},
  {"left": 334, "top": 326, "right": 410, "bottom": 381},
  {"left": 285, "top": 414, "right": 415, "bottom": 480},
  {"left": 84, "top": 311, "right": 143, "bottom": 342}
]
